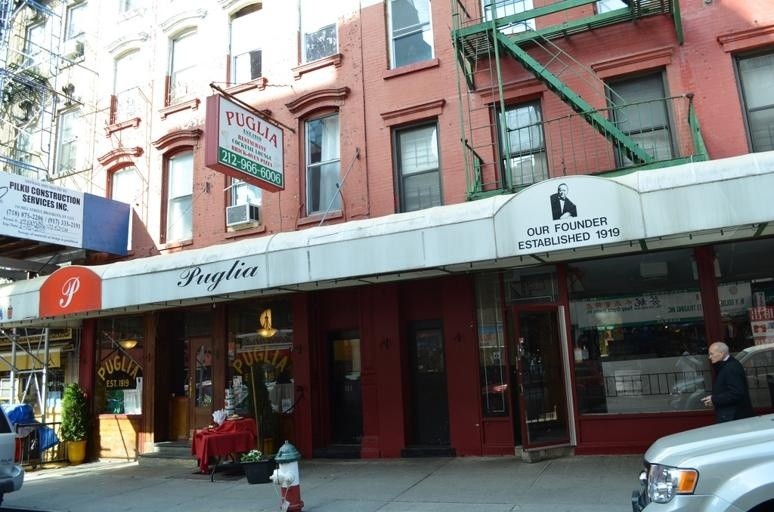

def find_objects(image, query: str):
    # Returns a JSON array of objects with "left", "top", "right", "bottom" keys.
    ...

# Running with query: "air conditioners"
[{"left": 226, "top": 203, "right": 260, "bottom": 227}]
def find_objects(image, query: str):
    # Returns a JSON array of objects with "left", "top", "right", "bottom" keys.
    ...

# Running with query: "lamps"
[
  {"left": 256, "top": 308, "right": 278, "bottom": 338},
  {"left": 118, "top": 340, "right": 138, "bottom": 350}
]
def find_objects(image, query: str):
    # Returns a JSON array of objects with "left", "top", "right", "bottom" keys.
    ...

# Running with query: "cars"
[
  {"left": 631, "top": 412, "right": 773, "bottom": 511},
  {"left": 0, "top": 403, "right": 25, "bottom": 505},
  {"left": 667, "top": 343, "right": 774, "bottom": 410}
]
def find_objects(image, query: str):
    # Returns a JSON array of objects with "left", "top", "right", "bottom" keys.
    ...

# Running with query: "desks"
[{"left": 192, "top": 418, "right": 257, "bottom": 482}]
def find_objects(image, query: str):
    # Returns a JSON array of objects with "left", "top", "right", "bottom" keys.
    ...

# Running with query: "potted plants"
[{"left": 61, "top": 382, "right": 89, "bottom": 465}]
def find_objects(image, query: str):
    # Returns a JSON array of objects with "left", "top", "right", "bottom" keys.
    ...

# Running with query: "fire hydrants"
[{"left": 269, "top": 440, "right": 304, "bottom": 512}]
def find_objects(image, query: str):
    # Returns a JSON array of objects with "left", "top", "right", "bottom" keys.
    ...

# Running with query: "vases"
[{"left": 240, "top": 456, "right": 276, "bottom": 484}]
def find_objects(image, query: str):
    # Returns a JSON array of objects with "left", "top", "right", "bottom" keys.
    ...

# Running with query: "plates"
[{"left": 226, "top": 417, "right": 245, "bottom": 420}]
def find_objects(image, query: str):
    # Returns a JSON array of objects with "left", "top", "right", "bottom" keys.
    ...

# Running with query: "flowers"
[{"left": 240, "top": 449, "right": 261, "bottom": 461}]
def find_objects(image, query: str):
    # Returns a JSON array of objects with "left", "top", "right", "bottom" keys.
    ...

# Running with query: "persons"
[
  {"left": 550, "top": 183, "right": 577, "bottom": 220},
  {"left": 700, "top": 342, "right": 754, "bottom": 422}
]
